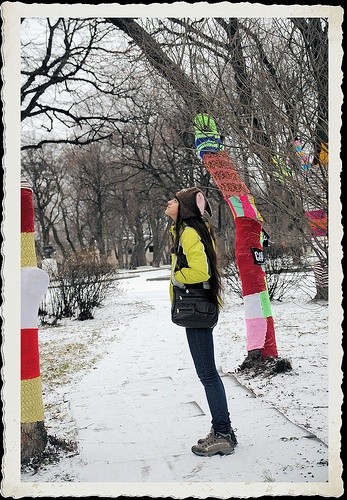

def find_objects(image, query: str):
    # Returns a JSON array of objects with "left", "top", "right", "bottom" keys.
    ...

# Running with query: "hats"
[{"left": 170, "top": 187, "right": 212, "bottom": 256}]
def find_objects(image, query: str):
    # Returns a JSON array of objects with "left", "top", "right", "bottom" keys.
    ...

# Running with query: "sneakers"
[{"left": 192, "top": 429, "right": 237, "bottom": 455}]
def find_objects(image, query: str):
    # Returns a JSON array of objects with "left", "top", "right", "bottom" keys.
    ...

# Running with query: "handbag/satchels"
[{"left": 171, "top": 286, "right": 218, "bottom": 329}]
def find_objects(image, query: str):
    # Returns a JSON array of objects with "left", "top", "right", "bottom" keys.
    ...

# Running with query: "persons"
[{"left": 166, "top": 186, "right": 236, "bottom": 457}]
